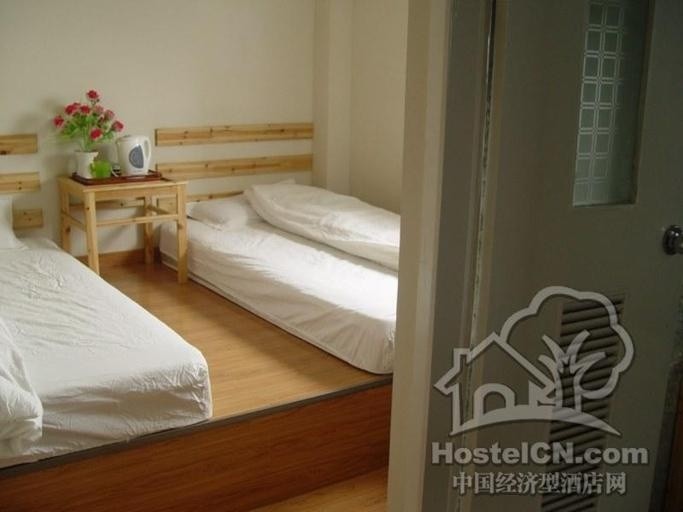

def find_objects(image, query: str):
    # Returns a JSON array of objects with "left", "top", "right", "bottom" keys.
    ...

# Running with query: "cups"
[{"left": 90, "top": 159, "right": 111, "bottom": 179}]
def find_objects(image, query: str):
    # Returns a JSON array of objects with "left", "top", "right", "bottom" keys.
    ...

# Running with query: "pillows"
[
  {"left": 182, "top": 193, "right": 262, "bottom": 232},
  {"left": 1, "top": 195, "right": 28, "bottom": 251}
]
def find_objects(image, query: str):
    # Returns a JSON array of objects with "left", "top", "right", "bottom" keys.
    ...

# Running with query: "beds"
[
  {"left": 0, "top": 135, "right": 210, "bottom": 468},
  {"left": 153, "top": 121, "right": 398, "bottom": 376}
]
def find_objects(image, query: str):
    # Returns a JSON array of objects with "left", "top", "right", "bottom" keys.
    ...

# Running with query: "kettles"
[{"left": 115, "top": 134, "right": 152, "bottom": 178}]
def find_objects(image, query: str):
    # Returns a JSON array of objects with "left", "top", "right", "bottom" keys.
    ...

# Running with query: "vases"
[{"left": 73, "top": 150, "right": 98, "bottom": 177}]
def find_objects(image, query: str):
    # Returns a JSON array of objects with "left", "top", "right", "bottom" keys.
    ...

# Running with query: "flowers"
[{"left": 49, "top": 90, "right": 123, "bottom": 150}]
